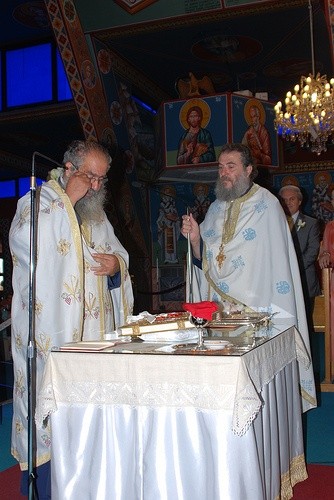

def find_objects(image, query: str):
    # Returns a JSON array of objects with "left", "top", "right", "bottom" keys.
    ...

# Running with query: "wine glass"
[{"left": 189, "top": 312, "right": 208, "bottom": 351}]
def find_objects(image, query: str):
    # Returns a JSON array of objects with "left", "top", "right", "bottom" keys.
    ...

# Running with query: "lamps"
[{"left": 273, "top": 0, "right": 334, "bottom": 155}]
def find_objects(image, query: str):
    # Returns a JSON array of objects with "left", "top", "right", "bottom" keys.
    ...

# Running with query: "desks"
[{"left": 49, "top": 320, "right": 307, "bottom": 500}]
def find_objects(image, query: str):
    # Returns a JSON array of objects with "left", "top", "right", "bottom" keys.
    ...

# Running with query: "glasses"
[{"left": 68, "top": 161, "right": 109, "bottom": 185}]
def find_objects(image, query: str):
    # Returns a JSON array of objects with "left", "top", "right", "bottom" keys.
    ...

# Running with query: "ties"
[{"left": 288, "top": 217, "right": 294, "bottom": 229}]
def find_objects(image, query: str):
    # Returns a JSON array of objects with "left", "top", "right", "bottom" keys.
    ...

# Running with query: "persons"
[
  {"left": 8, "top": 136, "right": 134, "bottom": 500},
  {"left": 317, "top": 182, "right": 334, "bottom": 269},
  {"left": 180, "top": 142, "right": 317, "bottom": 413},
  {"left": 277, "top": 185, "right": 320, "bottom": 409}
]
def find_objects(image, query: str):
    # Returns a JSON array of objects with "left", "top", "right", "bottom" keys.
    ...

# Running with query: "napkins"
[{"left": 183, "top": 301, "right": 218, "bottom": 321}]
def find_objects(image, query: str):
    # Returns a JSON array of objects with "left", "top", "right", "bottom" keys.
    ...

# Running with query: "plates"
[{"left": 203, "top": 340, "right": 230, "bottom": 350}]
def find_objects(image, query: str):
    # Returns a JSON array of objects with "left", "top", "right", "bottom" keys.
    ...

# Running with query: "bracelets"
[{"left": 321, "top": 251, "right": 330, "bottom": 256}]
